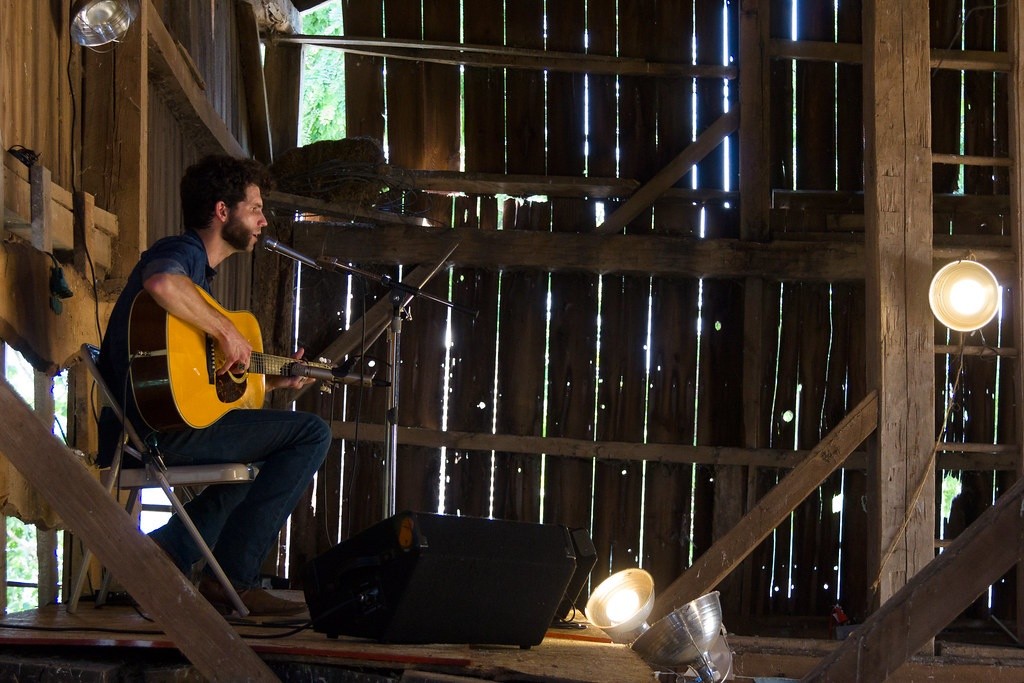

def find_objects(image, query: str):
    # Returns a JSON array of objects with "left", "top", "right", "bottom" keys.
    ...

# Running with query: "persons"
[{"left": 96, "top": 153, "right": 332, "bottom": 617}]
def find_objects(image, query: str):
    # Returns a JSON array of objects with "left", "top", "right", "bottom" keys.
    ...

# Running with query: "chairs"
[{"left": 66, "top": 343, "right": 252, "bottom": 617}]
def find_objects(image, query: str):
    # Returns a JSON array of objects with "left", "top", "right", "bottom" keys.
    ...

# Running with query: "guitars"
[{"left": 128, "top": 278, "right": 393, "bottom": 435}]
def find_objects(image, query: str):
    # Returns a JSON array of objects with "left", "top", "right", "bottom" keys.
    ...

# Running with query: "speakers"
[{"left": 299, "top": 510, "right": 578, "bottom": 650}]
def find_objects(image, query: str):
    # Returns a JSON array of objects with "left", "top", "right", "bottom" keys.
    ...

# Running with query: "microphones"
[{"left": 258, "top": 231, "right": 324, "bottom": 271}]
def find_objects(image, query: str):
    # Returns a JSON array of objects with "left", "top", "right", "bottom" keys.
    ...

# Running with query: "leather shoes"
[{"left": 199, "top": 574, "right": 307, "bottom": 616}]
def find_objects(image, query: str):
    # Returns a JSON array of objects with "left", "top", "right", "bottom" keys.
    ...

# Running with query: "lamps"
[
  {"left": 631, "top": 587, "right": 735, "bottom": 683},
  {"left": 931, "top": 259, "right": 1001, "bottom": 332},
  {"left": 583, "top": 568, "right": 657, "bottom": 640},
  {"left": 69, "top": 0, "right": 130, "bottom": 53}
]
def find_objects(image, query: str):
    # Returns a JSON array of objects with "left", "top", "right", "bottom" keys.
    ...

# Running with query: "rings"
[
  {"left": 233, "top": 362, "right": 245, "bottom": 370},
  {"left": 300, "top": 378, "right": 306, "bottom": 383}
]
